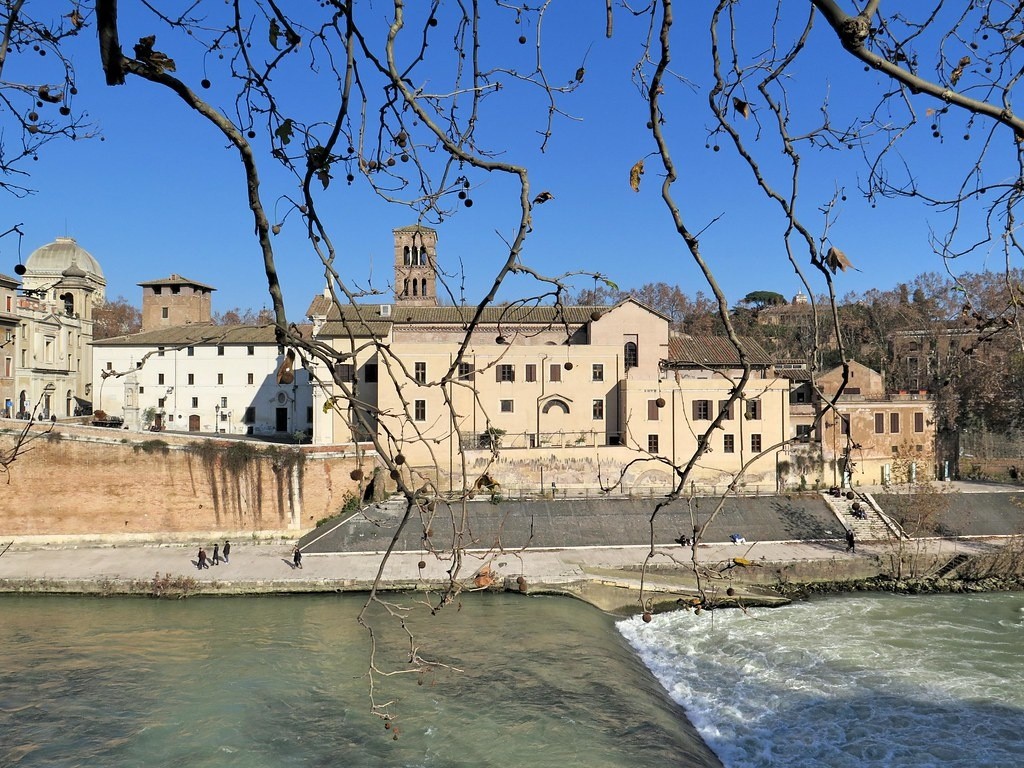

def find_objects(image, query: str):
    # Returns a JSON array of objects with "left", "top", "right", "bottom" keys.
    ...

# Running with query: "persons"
[
  {"left": 223, "top": 541, "right": 230, "bottom": 563},
  {"left": 732, "top": 533, "right": 746, "bottom": 546},
  {"left": 16, "top": 411, "right": 33, "bottom": 420},
  {"left": 195, "top": 548, "right": 209, "bottom": 570},
  {"left": 1009, "top": 466, "right": 1017, "bottom": 479},
  {"left": 38, "top": 413, "right": 42, "bottom": 422},
  {"left": 680, "top": 535, "right": 686, "bottom": 546},
  {"left": 74, "top": 406, "right": 86, "bottom": 416},
  {"left": 829, "top": 485, "right": 867, "bottom": 519},
  {"left": 293, "top": 545, "right": 303, "bottom": 569},
  {"left": 846, "top": 529, "right": 855, "bottom": 553},
  {"left": 51, "top": 412, "right": 56, "bottom": 422},
  {"left": 211, "top": 544, "right": 219, "bottom": 566}
]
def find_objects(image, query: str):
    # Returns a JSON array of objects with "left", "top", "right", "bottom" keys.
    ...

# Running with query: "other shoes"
[
  {"left": 207, "top": 566, "right": 209, "bottom": 569},
  {"left": 846, "top": 548, "right": 850, "bottom": 554},
  {"left": 211, "top": 564, "right": 214, "bottom": 566},
  {"left": 300, "top": 568, "right": 302, "bottom": 569},
  {"left": 853, "top": 551, "right": 856, "bottom": 554},
  {"left": 216, "top": 564, "right": 218, "bottom": 565}
]
